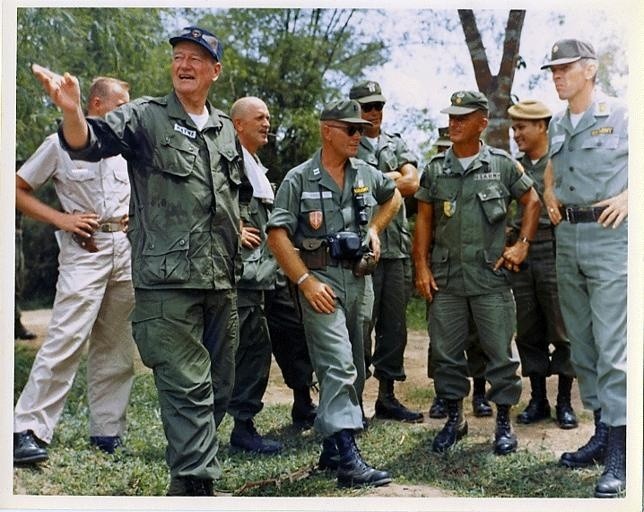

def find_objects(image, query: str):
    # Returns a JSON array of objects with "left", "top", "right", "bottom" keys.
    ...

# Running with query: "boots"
[
  {"left": 230, "top": 418, "right": 285, "bottom": 454},
  {"left": 560, "top": 408, "right": 610, "bottom": 469},
  {"left": 515, "top": 375, "right": 551, "bottom": 425},
  {"left": 15, "top": 317, "right": 37, "bottom": 341},
  {"left": 594, "top": 425, "right": 626, "bottom": 499},
  {"left": 492, "top": 404, "right": 517, "bottom": 454},
  {"left": 318, "top": 429, "right": 393, "bottom": 489},
  {"left": 351, "top": 376, "right": 427, "bottom": 431},
  {"left": 430, "top": 394, "right": 468, "bottom": 451},
  {"left": 473, "top": 378, "right": 493, "bottom": 418},
  {"left": 291, "top": 385, "right": 319, "bottom": 424},
  {"left": 555, "top": 371, "right": 578, "bottom": 428}
]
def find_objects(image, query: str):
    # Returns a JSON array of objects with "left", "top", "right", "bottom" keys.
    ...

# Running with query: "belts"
[
  {"left": 510, "top": 228, "right": 554, "bottom": 246},
  {"left": 559, "top": 203, "right": 607, "bottom": 224},
  {"left": 94, "top": 222, "right": 125, "bottom": 234}
]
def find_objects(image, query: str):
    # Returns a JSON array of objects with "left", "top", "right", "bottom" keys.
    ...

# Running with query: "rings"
[
  {"left": 550, "top": 209, "right": 554, "bottom": 212},
  {"left": 507, "top": 265, "right": 512, "bottom": 270}
]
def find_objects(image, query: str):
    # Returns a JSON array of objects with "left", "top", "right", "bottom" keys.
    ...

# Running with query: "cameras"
[{"left": 327, "top": 232, "right": 362, "bottom": 258}]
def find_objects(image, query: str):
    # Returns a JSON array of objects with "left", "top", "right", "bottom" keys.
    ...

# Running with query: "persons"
[
  {"left": 349, "top": 81, "right": 424, "bottom": 429},
  {"left": 230, "top": 96, "right": 321, "bottom": 452},
  {"left": 543, "top": 40, "right": 627, "bottom": 497},
  {"left": 414, "top": 92, "right": 542, "bottom": 455},
  {"left": 15, "top": 77, "right": 136, "bottom": 466},
  {"left": 428, "top": 126, "right": 492, "bottom": 418},
  {"left": 506, "top": 100, "right": 576, "bottom": 429},
  {"left": 31, "top": 26, "right": 253, "bottom": 495},
  {"left": 15, "top": 157, "right": 36, "bottom": 340},
  {"left": 266, "top": 99, "right": 402, "bottom": 488}
]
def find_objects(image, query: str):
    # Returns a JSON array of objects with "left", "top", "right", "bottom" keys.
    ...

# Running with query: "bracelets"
[
  {"left": 297, "top": 272, "right": 310, "bottom": 285},
  {"left": 517, "top": 236, "right": 532, "bottom": 245}
]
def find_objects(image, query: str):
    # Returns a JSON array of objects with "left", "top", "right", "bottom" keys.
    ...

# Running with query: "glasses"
[
  {"left": 361, "top": 103, "right": 384, "bottom": 112},
  {"left": 324, "top": 123, "right": 364, "bottom": 136}
]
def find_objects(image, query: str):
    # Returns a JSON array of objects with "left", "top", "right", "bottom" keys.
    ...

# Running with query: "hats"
[
  {"left": 169, "top": 26, "right": 224, "bottom": 62},
  {"left": 507, "top": 97, "right": 553, "bottom": 120},
  {"left": 319, "top": 99, "right": 374, "bottom": 126},
  {"left": 540, "top": 38, "right": 598, "bottom": 70},
  {"left": 440, "top": 90, "right": 489, "bottom": 116},
  {"left": 350, "top": 80, "right": 386, "bottom": 106}
]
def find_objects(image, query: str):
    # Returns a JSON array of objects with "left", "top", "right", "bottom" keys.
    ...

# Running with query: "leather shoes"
[{"left": 14, "top": 429, "right": 49, "bottom": 466}]
